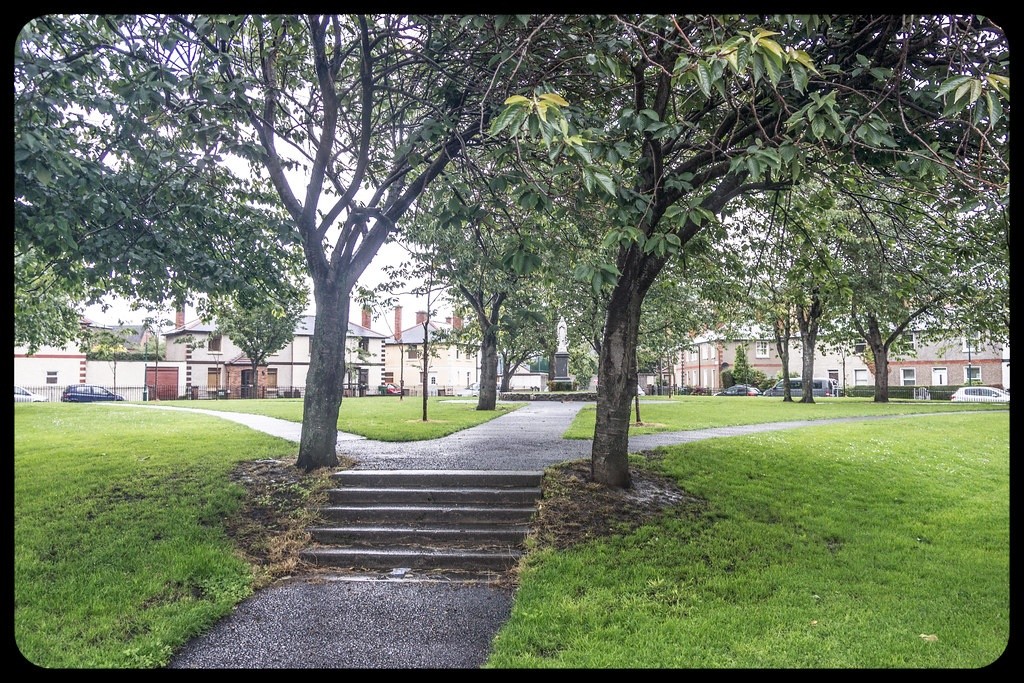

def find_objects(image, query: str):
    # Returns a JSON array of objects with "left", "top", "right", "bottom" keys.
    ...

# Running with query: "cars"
[
  {"left": 14, "top": 386, "right": 48, "bottom": 403},
  {"left": 951, "top": 386, "right": 1010, "bottom": 402},
  {"left": 384, "top": 383, "right": 401, "bottom": 395},
  {"left": 713, "top": 384, "right": 763, "bottom": 397},
  {"left": 638, "top": 384, "right": 646, "bottom": 396}
]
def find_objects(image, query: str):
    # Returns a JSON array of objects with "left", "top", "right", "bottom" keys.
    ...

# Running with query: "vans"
[
  {"left": 457, "top": 382, "right": 504, "bottom": 398},
  {"left": 61, "top": 384, "right": 124, "bottom": 402},
  {"left": 765, "top": 378, "right": 839, "bottom": 397}
]
